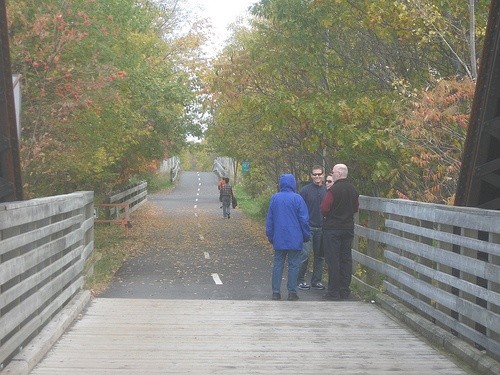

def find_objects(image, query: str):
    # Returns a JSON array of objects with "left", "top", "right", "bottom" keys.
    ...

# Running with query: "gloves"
[{"left": 268, "top": 236, "right": 273, "bottom": 244}]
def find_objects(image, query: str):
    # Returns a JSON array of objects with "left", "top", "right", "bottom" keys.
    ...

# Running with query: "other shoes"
[
  {"left": 322, "top": 292, "right": 352, "bottom": 301},
  {"left": 272, "top": 293, "right": 281, "bottom": 300},
  {"left": 287, "top": 293, "right": 299, "bottom": 301},
  {"left": 224, "top": 213, "right": 231, "bottom": 218},
  {"left": 220, "top": 205, "right": 223, "bottom": 208}
]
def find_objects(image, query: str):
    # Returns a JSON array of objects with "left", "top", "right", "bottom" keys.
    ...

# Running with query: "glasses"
[
  {"left": 326, "top": 180, "right": 332, "bottom": 183},
  {"left": 312, "top": 172, "right": 323, "bottom": 176}
]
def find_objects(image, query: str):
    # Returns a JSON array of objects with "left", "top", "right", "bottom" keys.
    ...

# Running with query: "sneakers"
[
  {"left": 296, "top": 282, "right": 310, "bottom": 290},
  {"left": 311, "top": 283, "right": 325, "bottom": 290}
]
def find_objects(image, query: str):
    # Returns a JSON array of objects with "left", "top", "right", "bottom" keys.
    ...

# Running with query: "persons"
[
  {"left": 320, "top": 164, "right": 359, "bottom": 301},
  {"left": 218, "top": 177, "right": 234, "bottom": 218},
  {"left": 324, "top": 173, "right": 335, "bottom": 191},
  {"left": 265, "top": 173, "right": 311, "bottom": 301},
  {"left": 298, "top": 164, "right": 331, "bottom": 290}
]
